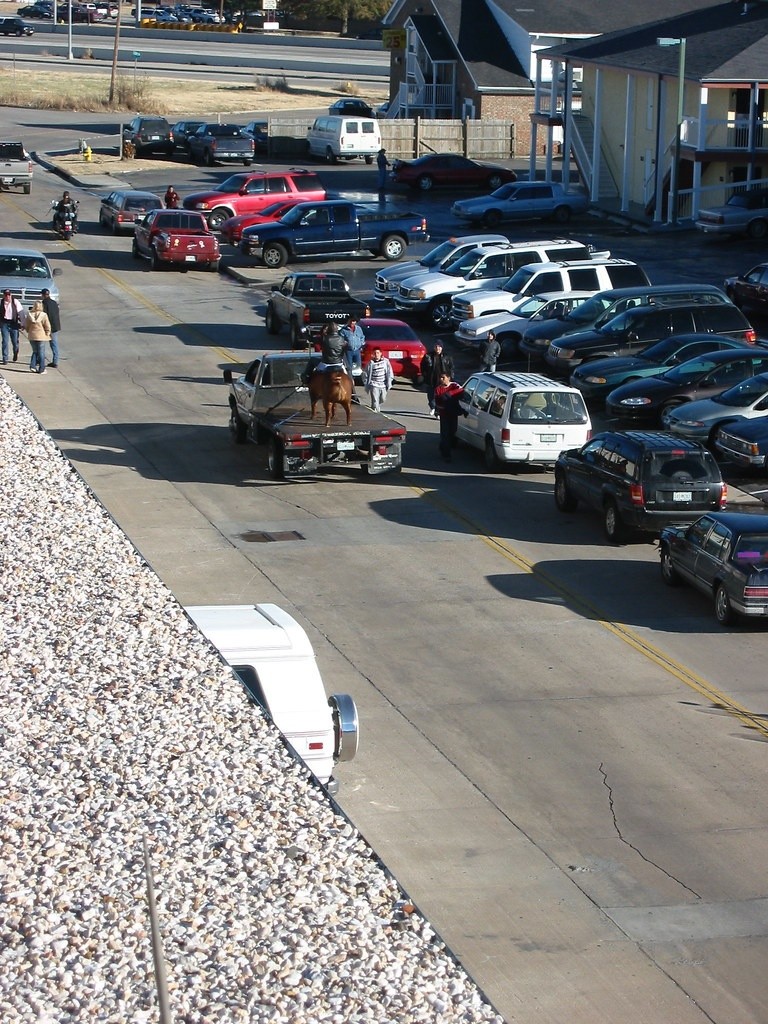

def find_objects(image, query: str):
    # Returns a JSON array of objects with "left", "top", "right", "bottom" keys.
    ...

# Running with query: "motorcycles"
[{"left": 50, "top": 199, "right": 80, "bottom": 241}]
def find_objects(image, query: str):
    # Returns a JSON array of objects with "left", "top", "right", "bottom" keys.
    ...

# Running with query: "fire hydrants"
[{"left": 82, "top": 146, "right": 93, "bottom": 163}]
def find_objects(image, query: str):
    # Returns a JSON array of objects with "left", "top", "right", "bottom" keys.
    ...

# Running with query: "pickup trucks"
[
  {"left": 222, "top": 352, "right": 406, "bottom": 481},
  {"left": 238, "top": 200, "right": 432, "bottom": 269},
  {"left": 130, "top": 208, "right": 223, "bottom": 273},
  {"left": 0, "top": 140, "right": 34, "bottom": 195}
]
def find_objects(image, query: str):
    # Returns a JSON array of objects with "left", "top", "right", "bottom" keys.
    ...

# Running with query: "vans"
[{"left": 306, "top": 115, "right": 384, "bottom": 165}]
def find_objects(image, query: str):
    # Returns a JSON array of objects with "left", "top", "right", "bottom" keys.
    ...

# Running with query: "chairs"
[
  {"left": 625, "top": 300, "right": 637, "bottom": 313},
  {"left": 524, "top": 391, "right": 548, "bottom": 414},
  {"left": 726, "top": 363, "right": 747, "bottom": 382}
]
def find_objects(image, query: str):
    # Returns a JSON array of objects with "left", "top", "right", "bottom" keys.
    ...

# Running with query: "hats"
[
  {"left": 432, "top": 339, "right": 444, "bottom": 348},
  {"left": 4, "top": 289, "right": 10, "bottom": 293},
  {"left": 349, "top": 314, "right": 359, "bottom": 322},
  {"left": 39, "top": 288, "right": 49, "bottom": 295}
]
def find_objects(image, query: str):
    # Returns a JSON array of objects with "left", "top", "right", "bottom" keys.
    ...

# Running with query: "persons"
[
  {"left": 313, "top": 323, "right": 328, "bottom": 352},
  {"left": 420, "top": 339, "right": 454, "bottom": 419},
  {"left": 0, "top": 289, "right": 25, "bottom": 364},
  {"left": 304, "top": 321, "right": 355, "bottom": 390},
  {"left": 24, "top": 300, "right": 51, "bottom": 375},
  {"left": 36, "top": 288, "right": 61, "bottom": 368},
  {"left": 475, "top": 396, "right": 506, "bottom": 435},
  {"left": 376, "top": 149, "right": 391, "bottom": 181},
  {"left": 362, "top": 347, "right": 394, "bottom": 413},
  {"left": 339, "top": 316, "right": 365, "bottom": 385},
  {"left": 52, "top": 191, "right": 79, "bottom": 233},
  {"left": 19, "top": 259, "right": 45, "bottom": 273},
  {"left": 164, "top": 185, "right": 180, "bottom": 209},
  {"left": 476, "top": 329, "right": 500, "bottom": 372},
  {"left": 434, "top": 371, "right": 465, "bottom": 456}
]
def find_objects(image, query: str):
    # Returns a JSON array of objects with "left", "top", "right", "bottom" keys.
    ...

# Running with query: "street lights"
[{"left": 656, "top": 34, "right": 686, "bottom": 231}]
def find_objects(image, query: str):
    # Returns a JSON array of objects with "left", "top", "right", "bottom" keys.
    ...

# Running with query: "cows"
[{"left": 309, "top": 370, "right": 352, "bottom": 428}]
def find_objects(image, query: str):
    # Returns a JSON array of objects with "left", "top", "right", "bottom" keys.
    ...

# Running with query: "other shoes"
[
  {"left": 3, "top": 359, "right": 7, "bottom": 365},
  {"left": 30, "top": 368, "right": 38, "bottom": 373},
  {"left": 429, "top": 409, "right": 435, "bottom": 416},
  {"left": 13, "top": 354, "right": 17, "bottom": 361},
  {"left": 48, "top": 363, "right": 57, "bottom": 367},
  {"left": 38, "top": 367, "right": 44, "bottom": 373},
  {"left": 36, "top": 359, "right": 40, "bottom": 364}
]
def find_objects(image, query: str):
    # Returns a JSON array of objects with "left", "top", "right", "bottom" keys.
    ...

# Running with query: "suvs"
[
  {"left": 171, "top": 120, "right": 211, "bottom": 148},
  {"left": 180, "top": 168, "right": 327, "bottom": 231},
  {"left": 553, "top": 429, "right": 728, "bottom": 543},
  {"left": 265, "top": 233, "right": 768, "bottom": 475},
  {"left": 180, "top": 602, "right": 359, "bottom": 800},
  {"left": 240, "top": 120, "right": 268, "bottom": 150},
  {"left": 187, "top": 122, "right": 256, "bottom": 168},
  {"left": 124, "top": 114, "right": 180, "bottom": 158}
]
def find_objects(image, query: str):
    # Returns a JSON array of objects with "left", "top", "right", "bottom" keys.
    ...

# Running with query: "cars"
[
  {"left": 0, "top": 0, "right": 231, "bottom": 38},
  {"left": 99, "top": 189, "right": 168, "bottom": 238},
  {"left": 0, "top": 247, "right": 63, "bottom": 320},
  {"left": 655, "top": 511, "right": 768, "bottom": 630},
  {"left": 693, "top": 187, "right": 768, "bottom": 240},
  {"left": 218, "top": 197, "right": 315, "bottom": 248},
  {"left": 387, "top": 153, "right": 519, "bottom": 195},
  {"left": 449, "top": 178, "right": 592, "bottom": 227},
  {"left": 329, "top": 99, "right": 391, "bottom": 121}
]
